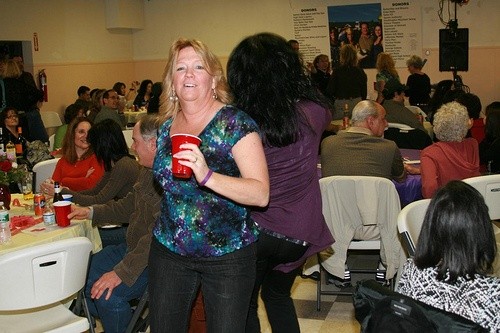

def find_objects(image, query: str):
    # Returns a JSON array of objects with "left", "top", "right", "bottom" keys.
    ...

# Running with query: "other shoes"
[
  {"left": 375, "top": 268, "right": 391, "bottom": 287},
  {"left": 325, "top": 269, "right": 353, "bottom": 288}
]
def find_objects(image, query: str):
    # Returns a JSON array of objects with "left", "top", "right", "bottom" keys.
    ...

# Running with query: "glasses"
[{"left": 7, "top": 115, "right": 18, "bottom": 119}]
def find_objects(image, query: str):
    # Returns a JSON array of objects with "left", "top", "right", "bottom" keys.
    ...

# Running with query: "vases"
[{"left": 0, "top": 182, "right": 11, "bottom": 211}]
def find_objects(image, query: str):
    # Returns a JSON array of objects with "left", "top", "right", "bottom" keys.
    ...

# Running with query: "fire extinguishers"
[{"left": 39, "top": 68, "right": 49, "bottom": 103}]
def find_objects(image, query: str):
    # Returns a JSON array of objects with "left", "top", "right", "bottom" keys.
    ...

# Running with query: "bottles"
[
  {"left": 0, "top": 201, "right": 12, "bottom": 244},
  {"left": 21, "top": 166, "right": 34, "bottom": 199},
  {"left": 5, "top": 141, "right": 16, "bottom": 161},
  {"left": 53, "top": 181, "right": 63, "bottom": 203},
  {"left": 343, "top": 104, "right": 350, "bottom": 128},
  {"left": 0, "top": 127, "right": 4, "bottom": 152},
  {"left": 15, "top": 127, "right": 28, "bottom": 164}
]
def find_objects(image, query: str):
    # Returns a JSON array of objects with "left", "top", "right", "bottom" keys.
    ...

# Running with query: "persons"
[
  {"left": 330, "top": 22, "right": 383, "bottom": 69},
  {"left": 0, "top": 32, "right": 500, "bottom": 333}
]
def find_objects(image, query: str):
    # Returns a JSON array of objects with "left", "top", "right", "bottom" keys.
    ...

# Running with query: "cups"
[
  {"left": 53, "top": 200, "right": 71, "bottom": 227},
  {"left": 61, "top": 194, "right": 73, "bottom": 202},
  {"left": 170, "top": 134, "right": 202, "bottom": 179}
]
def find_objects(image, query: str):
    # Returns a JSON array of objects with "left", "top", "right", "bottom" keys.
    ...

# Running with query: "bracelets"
[{"left": 199, "top": 170, "right": 214, "bottom": 187}]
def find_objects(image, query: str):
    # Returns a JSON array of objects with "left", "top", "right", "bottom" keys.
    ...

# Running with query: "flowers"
[{"left": 0, "top": 148, "right": 30, "bottom": 185}]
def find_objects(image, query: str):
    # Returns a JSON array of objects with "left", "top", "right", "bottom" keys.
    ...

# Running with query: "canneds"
[{"left": 33, "top": 193, "right": 46, "bottom": 215}]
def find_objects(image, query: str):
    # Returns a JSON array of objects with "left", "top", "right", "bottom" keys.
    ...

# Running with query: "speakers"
[{"left": 438, "top": 28, "right": 469, "bottom": 72}]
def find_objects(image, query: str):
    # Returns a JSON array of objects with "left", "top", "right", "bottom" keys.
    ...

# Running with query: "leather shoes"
[{"left": 300, "top": 271, "right": 319, "bottom": 280}]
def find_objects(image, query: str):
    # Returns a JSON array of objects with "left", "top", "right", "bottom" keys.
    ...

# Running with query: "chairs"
[
  {"left": 42, "top": 111, "right": 62, "bottom": 150},
  {"left": 0, "top": 236, "right": 95, "bottom": 333},
  {"left": 123, "top": 130, "right": 135, "bottom": 149},
  {"left": 397, "top": 197, "right": 432, "bottom": 254},
  {"left": 300, "top": 175, "right": 407, "bottom": 310},
  {"left": 461, "top": 173, "right": 500, "bottom": 222},
  {"left": 32, "top": 157, "right": 60, "bottom": 192}
]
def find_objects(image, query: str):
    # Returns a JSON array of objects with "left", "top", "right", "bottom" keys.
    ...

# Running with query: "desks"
[
  {"left": 0, "top": 194, "right": 103, "bottom": 312},
  {"left": 122, "top": 111, "right": 146, "bottom": 128},
  {"left": 391, "top": 148, "right": 422, "bottom": 204}
]
